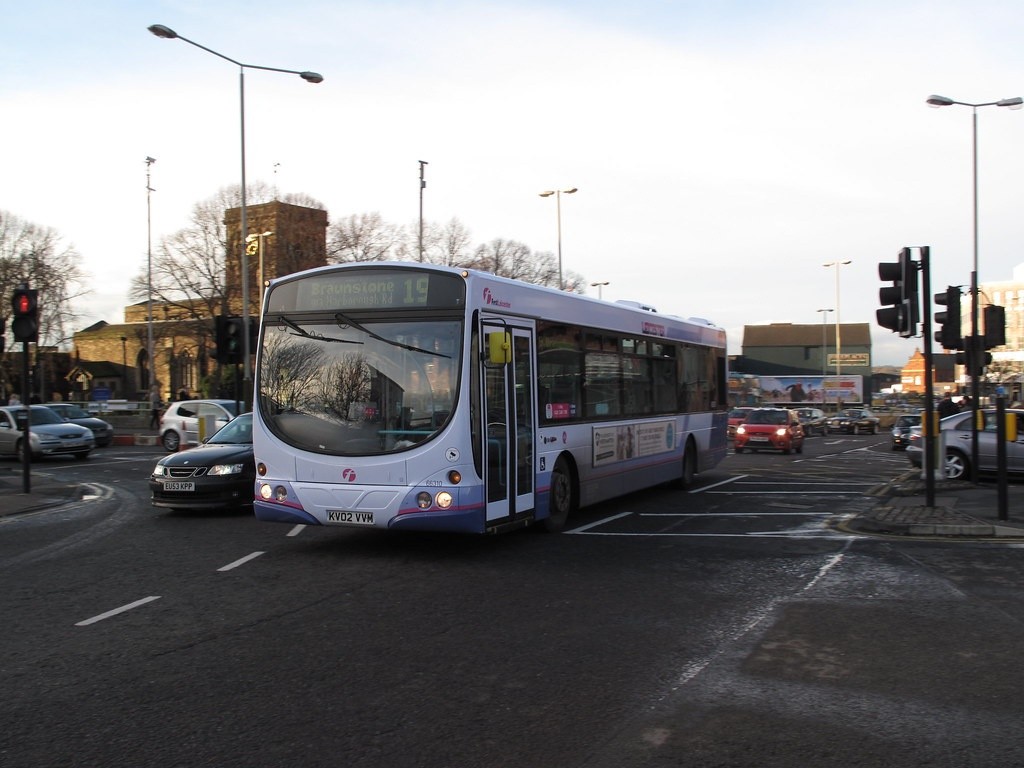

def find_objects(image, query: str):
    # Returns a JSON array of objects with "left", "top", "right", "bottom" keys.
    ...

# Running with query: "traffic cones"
[{"left": 178, "top": 420, "right": 189, "bottom": 451}]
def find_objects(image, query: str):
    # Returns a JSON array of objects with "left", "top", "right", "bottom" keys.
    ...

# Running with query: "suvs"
[{"left": 159, "top": 397, "right": 247, "bottom": 453}]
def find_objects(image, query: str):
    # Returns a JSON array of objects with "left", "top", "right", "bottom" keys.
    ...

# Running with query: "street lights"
[
  {"left": 537, "top": 187, "right": 578, "bottom": 292},
  {"left": 248, "top": 231, "right": 275, "bottom": 322},
  {"left": 818, "top": 309, "right": 835, "bottom": 412},
  {"left": 120, "top": 336, "right": 129, "bottom": 400},
  {"left": 590, "top": 281, "right": 609, "bottom": 299},
  {"left": 926, "top": 95, "right": 1023, "bottom": 485},
  {"left": 822, "top": 260, "right": 853, "bottom": 411},
  {"left": 146, "top": 22, "right": 328, "bottom": 431}
]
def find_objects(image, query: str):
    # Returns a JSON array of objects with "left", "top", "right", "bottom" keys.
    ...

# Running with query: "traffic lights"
[
  {"left": 955, "top": 334, "right": 972, "bottom": 376},
  {"left": 219, "top": 318, "right": 244, "bottom": 365},
  {"left": 873, "top": 248, "right": 921, "bottom": 339},
  {"left": 975, "top": 334, "right": 994, "bottom": 376},
  {"left": 248, "top": 318, "right": 259, "bottom": 355},
  {"left": 933, "top": 285, "right": 962, "bottom": 351},
  {"left": 207, "top": 314, "right": 223, "bottom": 363},
  {"left": 10, "top": 287, "right": 40, "bottom": 343}
]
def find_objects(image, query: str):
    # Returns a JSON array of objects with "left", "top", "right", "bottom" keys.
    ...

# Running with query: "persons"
[
  {"left": 2, "top": 393, "right": 21, "bottom": 405},
  {"left": 149, "top": 385, "right": 162, "bottom": 429},
  {"left": 958, "top": 396, "right": 974, "bottom": 412},
  {"left": 937, "top": 393, "right": 960, "bottom": 418}
]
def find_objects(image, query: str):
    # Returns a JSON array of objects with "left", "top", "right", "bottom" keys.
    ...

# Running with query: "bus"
[{"left": 251, "top": 259, "right": 728, "bottom": 538}]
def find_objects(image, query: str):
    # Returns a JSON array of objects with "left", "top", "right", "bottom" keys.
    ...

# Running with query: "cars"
[
  {"left": 904, "top": 407, "right": 1024, "bottom": 481},
  {"left": 889, "top": 414, "right": 923, "bottom": 452},
  {"left": 36, "top": 402, "right": 115, "bottom": 447},
  {"left": 826, "top": 408, "right": 881, "bottom": 435},
  {"left": 148, "top": 411, "right": 256, "bottom": 508},
  {"left": 733, "top": 408, "right": 805, "bottom": 454},
  {"left": 790, "top": 406, "right": 829, "bottom": 438},
  {"left": 727, "top": 406, "right": 757, "bottom": 439},
  {"left": 0, "top": 404, "right": 97, "bottom": 463}
]
